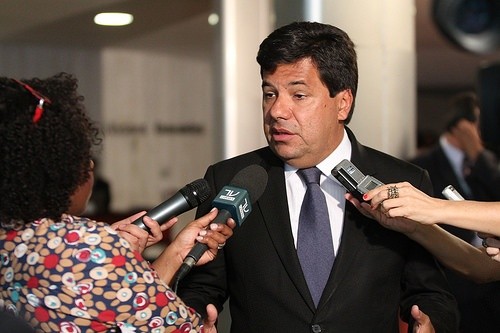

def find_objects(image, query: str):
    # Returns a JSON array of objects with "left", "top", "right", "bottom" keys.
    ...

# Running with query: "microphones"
[
  {"left": 175, "top": 164, "right": 269, "bottom": 279},
  {"left": 130, "top": 177, "right": 211, "bottom": 234}
]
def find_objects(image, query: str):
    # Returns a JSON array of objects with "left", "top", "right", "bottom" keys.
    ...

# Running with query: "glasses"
[{"left": 10, "top": 77, "right": 51, "bottom": 126}]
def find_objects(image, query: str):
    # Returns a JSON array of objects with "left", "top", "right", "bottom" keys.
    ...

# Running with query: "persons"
[
  {"left": 344, "top": 88, "right": 500, "bottom": 282},
  {"left": 0, "top": 71, "right": 217, "bottom": 333},
  {"left": 170, "top": 21, "right": 458, "bottom": 333}
]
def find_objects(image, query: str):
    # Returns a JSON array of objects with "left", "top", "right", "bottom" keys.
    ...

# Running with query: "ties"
[{"left": 297, "top": 168, "right": 335, "bottom": 308}]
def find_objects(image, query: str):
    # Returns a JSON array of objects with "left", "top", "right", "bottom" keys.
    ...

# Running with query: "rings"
[
  {"left": 388, "top": 187, "right": 399, "bottom": 198},
  {"left": 218, "top": 242, "right": 226, "bottom": 249}
]
